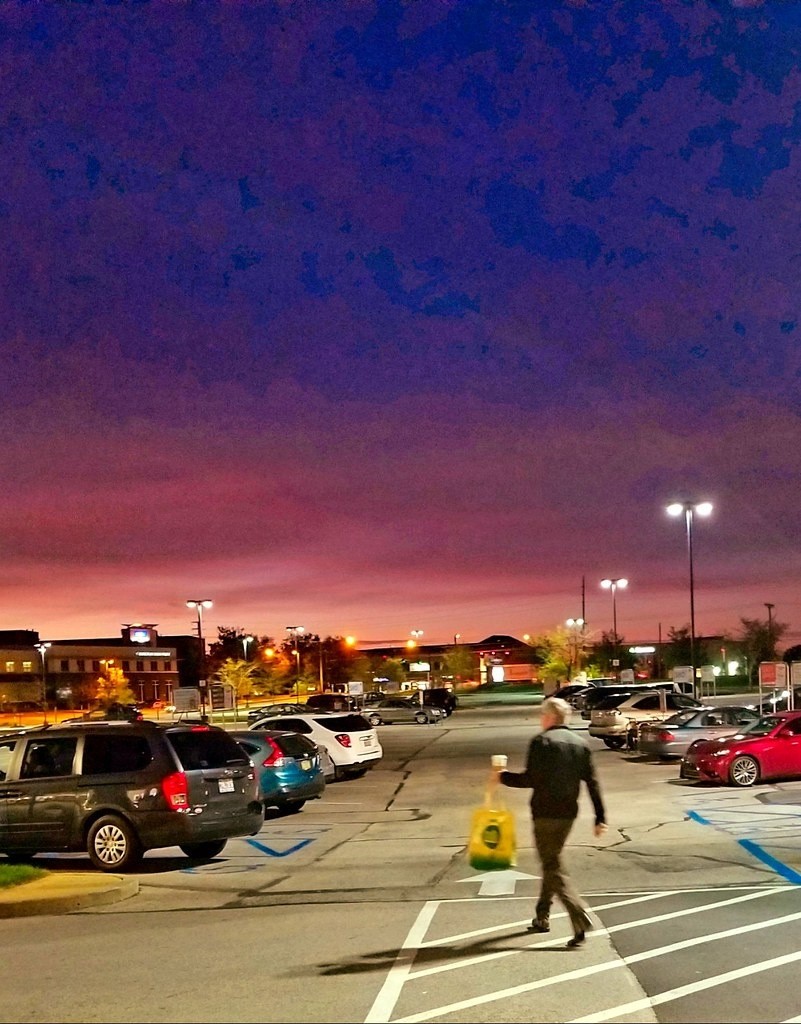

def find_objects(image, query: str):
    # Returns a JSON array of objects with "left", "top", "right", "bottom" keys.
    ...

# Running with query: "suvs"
[
  {"left": 307, "top": 690, "right": 385, "bottom": 713},
  {"left": 247, "top": 711, "right": 384, "bottom": 781},
  {"left": 0, "top": 716, "right": 266, "bottom": 872},
  {"left": 407, "top": 688, "right": 460, "bottom": 717}
]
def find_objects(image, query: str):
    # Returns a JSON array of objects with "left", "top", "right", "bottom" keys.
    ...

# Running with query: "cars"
[
  {"left": 544, "top": 677, "right": 702, "bottom": 720},
  {"left": 226, "top": 730, "right": 326, "bottom": 815},
  {"left": 360, "top": 697, "right": 447, "bottom": 726},
  {"left": 61, "top": 708, "right": 110, "bottom": 724},
  {"left": 755, "top": 685, "right": 801, "bottom": 714},
  {"left": 680, "top": 708, "right": 801, "bottom": 787},
  {"left": 315, "top": 744, "right": 336, "bottom": 783},
  {"left": 588, "top": 690, "right": 707, "bottom": 750},
  {"left": 638, "top": 706, "right": 773, "bottom": 757},
  {"left": 247, "top": 702, "right": 330, "bottom": 728}
]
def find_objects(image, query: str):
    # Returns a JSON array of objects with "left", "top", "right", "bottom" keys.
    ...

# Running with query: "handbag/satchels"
[{"left": 470, "top": 781, "right": 517, "bottom": 871}]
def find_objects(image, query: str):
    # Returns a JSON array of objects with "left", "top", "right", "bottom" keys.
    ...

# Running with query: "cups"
[{"left": 490, "top": 755, "right": 508, "bottom": 771}]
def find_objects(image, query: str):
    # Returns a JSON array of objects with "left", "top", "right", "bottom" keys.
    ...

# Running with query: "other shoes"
[
  {"left": 568, "top": 931, "right": 586, "bottom": 947},
  {"left": 532, "top": 918, "right": 550, "bottom": 932}
]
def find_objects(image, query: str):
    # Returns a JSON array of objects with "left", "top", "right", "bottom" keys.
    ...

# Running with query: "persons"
[
  {"left": 491, "top": 696, "right": 610, "bottom": 947},
  {"left": 27, "top": 744, "right": 72, "bottom": 779}
]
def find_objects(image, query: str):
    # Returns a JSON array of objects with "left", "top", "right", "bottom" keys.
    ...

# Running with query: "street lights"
[
  {"left": 666, "top": 502, "right": 714, "bottom": 701},
  {"left": 601, "top": 578, "right": 629, "bottom": 648},
  {"left": 99, "top": 660, "right": 114, "bottom": 670},
  {"left": 567, "top": 617, "right": 585, "bottom": 664},
  {"left": 454, "top": 634, "right": 460, "bottom": 644},
  {"left": 286, "top": 626, "right": 305, "bottom": 657},
  {"left": 242, "top": 636, "right": 254, "bottom": 709},
  {"left": 411, "top": 629, "right": 423, "bottom": 646},
  {"left": 185, "top": 598, "right": 213, "bottom": 723},
  {"left": 291, "top": 649, "right": 299, "bottom": 704},
  {"left": 33, "top": 643, "right": 51, "bottom": 725}
]
